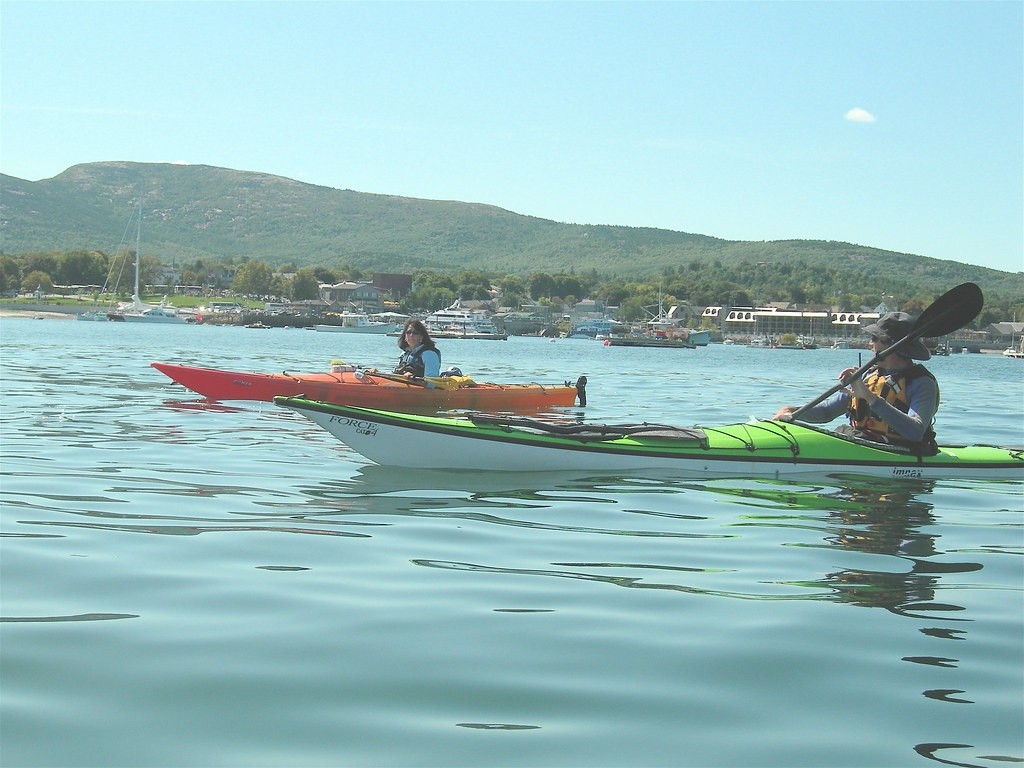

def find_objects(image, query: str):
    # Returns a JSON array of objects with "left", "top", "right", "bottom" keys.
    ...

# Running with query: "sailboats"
[{"left": 76, "top": 202, "right": 190, "bottom": 324}]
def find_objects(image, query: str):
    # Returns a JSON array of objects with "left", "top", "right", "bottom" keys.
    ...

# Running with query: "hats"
[{"left": 861, "top": 311, "right": 931, "bottom": 360}]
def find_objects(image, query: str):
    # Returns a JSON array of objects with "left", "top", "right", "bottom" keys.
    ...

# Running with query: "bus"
[
  {"left": 263, "top": 302, "right": 297, "bottom": 316},
  {"left": 210, "top": 302, "right": 244, "bottom": 314}
]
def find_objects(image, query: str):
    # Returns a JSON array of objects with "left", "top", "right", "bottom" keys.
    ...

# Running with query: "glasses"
[
  {"left": 871, "top": 334, "right": 879, "bottom": 342},
  {"left": 405, "top": 330, "right": 421, "bottom": 335}
]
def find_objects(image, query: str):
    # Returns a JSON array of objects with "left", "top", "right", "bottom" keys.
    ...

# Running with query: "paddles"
[
  {"left": 331, "top": 359, "right": 459, "bottom": 392},
  {"left": 781, "top": 282, "right": 985, "bottom": 425}
]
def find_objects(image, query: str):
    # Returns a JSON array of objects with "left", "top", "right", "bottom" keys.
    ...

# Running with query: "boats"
[
  {"left": 272, "top": 390, "right": 1024, "bottom": 478},
  {"left": 387, "top": 309, "right": 508, "bottom": 340},
  {"left": 312, "top": 311, "right": 396, "bottom": 335},
  {"left": 687, "top": 328, "right": 709, "bottom": 346},
  {"left": 1003, "top": 311, "right": 1024, "bottom": 360},
  {"left": 149, "top": 360, "right": 588, "bottom": 415}
]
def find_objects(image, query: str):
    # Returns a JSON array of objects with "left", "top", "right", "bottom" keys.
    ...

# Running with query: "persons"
[
  {"left": 368, "top": 319, "right": 442, "bottom": 389},
  {"left": 772, "top": 312, "right": 939, "bottom": 442}
]
